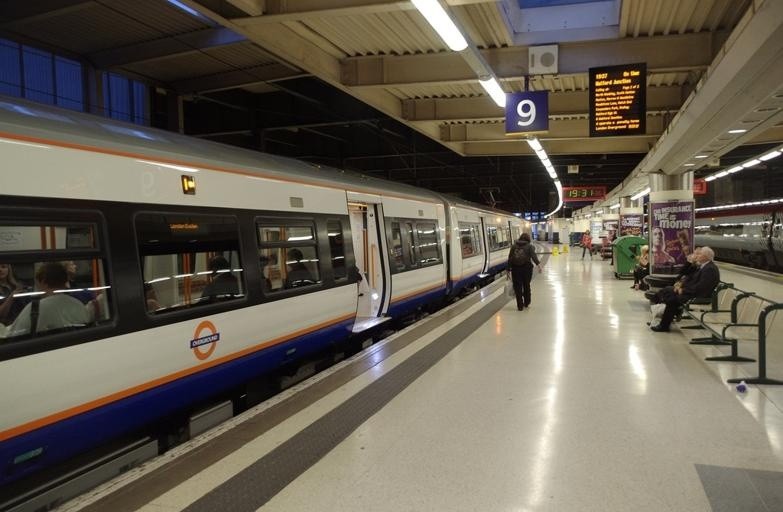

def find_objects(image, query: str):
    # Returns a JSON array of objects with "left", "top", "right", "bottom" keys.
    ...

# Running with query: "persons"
[
  {"left": 505, "top": 232, "right": 542, "bottom": 310},
  {"left": 582, "top": 229, "right": 594, "bottom": 260},
  {"left": 259, "top": 249, "right": 316, "bottom": 294},
  {"left": 144, "top": 280, "right": 161, "bottom": 311},
  {"left": 633, "top": 245, "right": 649, "bottom": 290},
  {"left": 200, "top": 256, "right": 239, "bottom": 301},
  {"left": 651, "top": 226, "right": 677, "bottom": 262},
  {"left": 2, "top": 262, "right": 105, "bottom": 335},
  {"left": 643, "top": 246, "right": 719, "bottom": 332}
]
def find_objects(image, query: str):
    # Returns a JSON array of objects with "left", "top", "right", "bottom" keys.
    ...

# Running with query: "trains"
[
  {"left": 640, "top": 199, "right": 783, "bottom": 274},
  {"left": 0, "top": 93, "right": 532, "bottom": 495}
]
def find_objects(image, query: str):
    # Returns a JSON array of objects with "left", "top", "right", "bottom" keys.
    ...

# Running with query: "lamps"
[{"left": 408, "top": 0, "right": 566, "bottom": 219}]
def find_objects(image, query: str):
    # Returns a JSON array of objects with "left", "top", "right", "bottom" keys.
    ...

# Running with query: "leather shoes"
[{"left": 650, "top": 325, "right": 670, "bottom": 332}]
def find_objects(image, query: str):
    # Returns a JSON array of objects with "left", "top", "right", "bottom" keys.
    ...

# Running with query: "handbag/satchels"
[
  {"left": 504, "top": 280, "right": 516, "bottom": 299},
  {"left": 648, "top": 303, "right": 666, "bottom": 331}
]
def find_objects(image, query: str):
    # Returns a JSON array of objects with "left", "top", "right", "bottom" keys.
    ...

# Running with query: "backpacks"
[{"left": 513, "top": 244, "right": 532, "bottom": 267}]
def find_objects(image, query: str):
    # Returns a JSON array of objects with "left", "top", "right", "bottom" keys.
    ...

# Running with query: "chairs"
[{"left": 681, "top": 282, "right": 782, "bottom": 385}]
